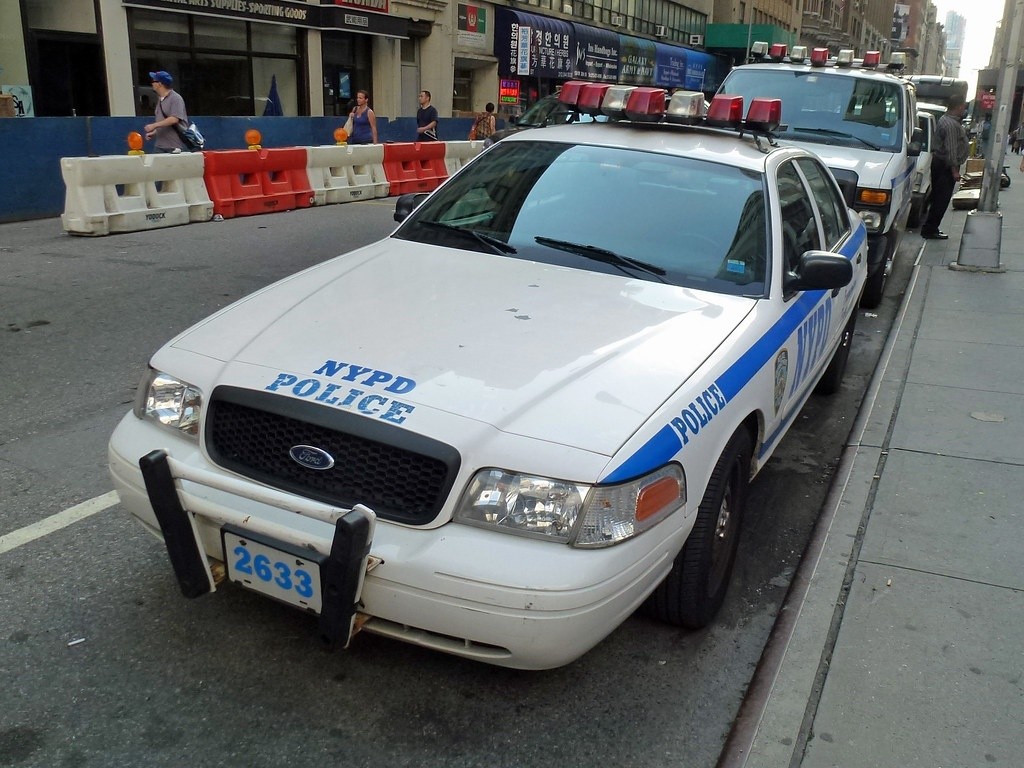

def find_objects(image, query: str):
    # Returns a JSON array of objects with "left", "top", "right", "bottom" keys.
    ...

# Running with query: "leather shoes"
[
  {"left": 923, "top": 234, "right": 948, "bottom": 240},
  {"left": 920, "top": 230, "right": 944, "bottom": 235}
]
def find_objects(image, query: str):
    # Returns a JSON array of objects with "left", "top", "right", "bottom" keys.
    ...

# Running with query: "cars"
[
  {"left": 481, "top": 88, "right": 710, "bottom": 153},
  {"left": 107, "top": 78, "right": 870, "bottom": 671},
  {"left": 834, "top": 101, "right": 949, "bottom": 228},
  {"left": 229, "top": 96, "right": 279, "bottom": 115}
]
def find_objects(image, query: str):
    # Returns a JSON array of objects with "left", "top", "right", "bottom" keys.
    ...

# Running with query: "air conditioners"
[
  {"left": 655, "top": 25, "right": 669, "bottom": 37},
  {"left": 612, "top": 17, "right": 623, "bottom": 26},
  {"left": 690, "top": 35, "right": 704, "bottom": 45}
]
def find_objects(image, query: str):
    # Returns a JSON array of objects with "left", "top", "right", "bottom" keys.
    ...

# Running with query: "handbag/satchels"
[
  {"left": 469, "top": 126, "right": 478, "bottom": 141},
  {"left": 183, "top": 120, "right": 206, "bottom": 153},
  {"left": 343, "top": 109, "right": 355, "bottom": 137}
]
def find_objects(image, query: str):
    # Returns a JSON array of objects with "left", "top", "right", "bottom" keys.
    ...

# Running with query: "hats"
[{"left": 149, "top": 70, "right": 173, "bottom": 88}]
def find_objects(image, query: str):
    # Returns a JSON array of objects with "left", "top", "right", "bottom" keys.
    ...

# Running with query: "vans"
[
  {"left": 896, "top": 75, "right": 968, "bottom": 112},
  {"left": 705, "top": 41, "right": 925, "bottom": 303}
]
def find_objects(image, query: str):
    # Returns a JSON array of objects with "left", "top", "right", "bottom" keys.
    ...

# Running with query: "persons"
[
  {"left": 144, "top": 71, "right": 193, "bottom": 193},
  {"left": 351, "top": 90, "right": 377, "bottom": 144},
  {"left": 468, "top": 102, "right": 496, "bottom": 141},
  {"left": 983, "top": 115, "right": 1024, "bottom": 172},
  {"left": 920, "top": 95, "right": 969, "bottom": 240},
  {"left": 416, "top": 90, "right": 438, "bottom": 142}
]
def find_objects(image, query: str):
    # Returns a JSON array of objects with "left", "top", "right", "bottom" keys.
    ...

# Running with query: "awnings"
[{"left": 498, "top": 9, "right": 719, "bottom": 92}]
